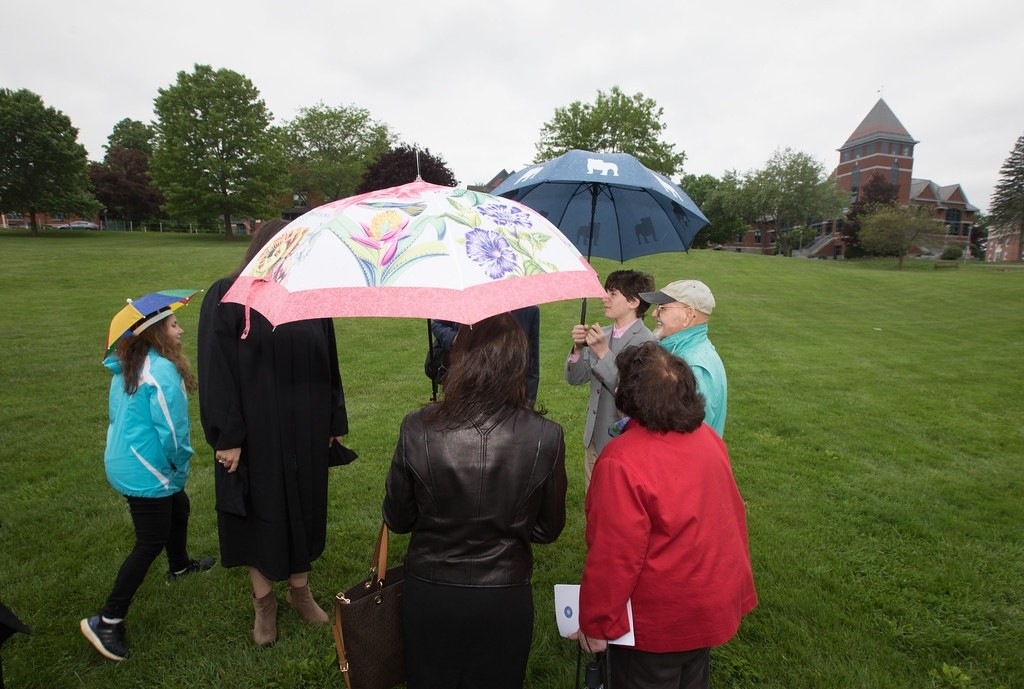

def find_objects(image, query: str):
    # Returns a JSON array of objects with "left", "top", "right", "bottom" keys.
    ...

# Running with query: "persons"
[
  {"left": 639, "top": 277, "right": 728, "bottom": 440},
  {"left": 563, "top": 268, "right": 657, "bottom": 492},
  {"left": 79, "top": 307, "right": 221, "bottom": 665},
  {"left": 196, "top": 219, "right": 349, "bottom": 650},
  {"left": 426, "top": 301, "right": 546, "bottom": 411},
  {"left": 383, "top": 309, "right": 569, "bottom": 689},
  {"left": 574, "top": 341, "right": 763, "bottom": 688}
]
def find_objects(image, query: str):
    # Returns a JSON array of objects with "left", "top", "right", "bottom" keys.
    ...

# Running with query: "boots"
[
  {"left": 250, "top": 592, "right": 277, "bottom": 645},
  {"left": 286, "top": 576, "right": 329, "bottom": 626}
]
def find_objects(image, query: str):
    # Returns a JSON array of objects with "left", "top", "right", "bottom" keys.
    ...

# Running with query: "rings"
[{"left": 218, "top": 459, "right": 224, "bottom": 464}]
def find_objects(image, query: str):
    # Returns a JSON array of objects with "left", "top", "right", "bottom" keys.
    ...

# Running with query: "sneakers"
[
  {"left": 164, "top": 558, "right": 217, "bottom": 586},
  {"left": 80, "top": 609, "right": 131, "bottom": 660}
]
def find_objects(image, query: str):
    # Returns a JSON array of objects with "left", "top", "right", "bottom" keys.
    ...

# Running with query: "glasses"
[{"left": 656, "top": 304, "right": 696, "bottom": 319}]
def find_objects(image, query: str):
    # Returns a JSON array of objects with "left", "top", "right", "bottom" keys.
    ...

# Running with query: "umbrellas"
[
  {"left": 103, "top": 289, "right": 200, "bottom": 361},
  {"left": 221, "top": 171, "right": 612, "bottom": 401},
  {"left": 484, "top": 153, "right": 711, "bottom": 263}
]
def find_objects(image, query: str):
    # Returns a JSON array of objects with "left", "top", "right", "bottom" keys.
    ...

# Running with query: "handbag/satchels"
[
  {"left": 425, "top": 336, "right": 453, "bottom": 385},
  {"left": 334, "top": 521, "right": 405, "bottom": 689}
]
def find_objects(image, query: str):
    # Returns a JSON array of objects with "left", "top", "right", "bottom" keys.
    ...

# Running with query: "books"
[{"left": 554, "top": 583, "right": 635, "bottom": 646}]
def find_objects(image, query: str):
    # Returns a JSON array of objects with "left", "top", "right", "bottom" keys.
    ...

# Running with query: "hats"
[{"left": 638, "top": 280, "right": 715, "bottom": 316}]
